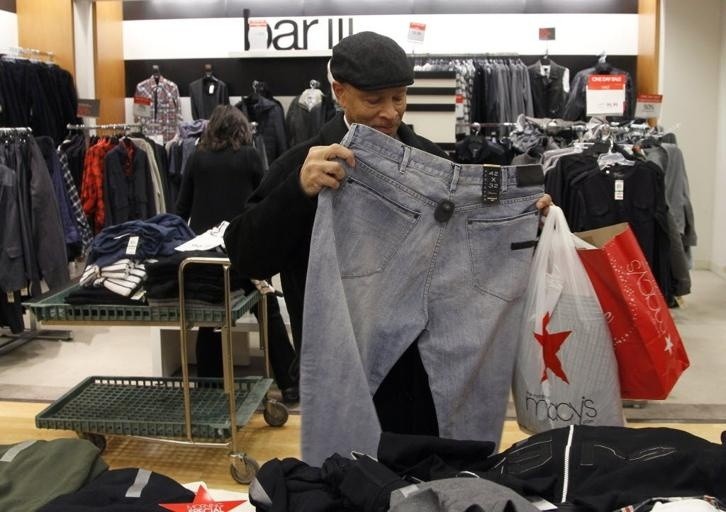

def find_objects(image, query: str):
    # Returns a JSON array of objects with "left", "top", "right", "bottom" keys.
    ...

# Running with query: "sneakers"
[{"left": 281, "top": 385, "right": 298, "bottom": 402}]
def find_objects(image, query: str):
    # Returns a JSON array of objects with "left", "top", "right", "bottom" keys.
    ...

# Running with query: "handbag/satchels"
[{"left": 574, "top": 223, "right": 689, "bottom": 401}]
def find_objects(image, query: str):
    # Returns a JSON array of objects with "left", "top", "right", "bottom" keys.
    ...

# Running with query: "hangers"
[
  {"left": 0, "top": 45, "right": 152, "bottom": 144},
  {"left": 467, "top": 113, "right": 677, "bottom": 169},
  {"left": 407, "top": 49, "right": 611, "bottom": 75}
]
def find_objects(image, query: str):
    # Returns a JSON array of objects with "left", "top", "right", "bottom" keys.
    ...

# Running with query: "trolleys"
[{"left": 22, "top": 256, "right": 288, "bottom": 484}]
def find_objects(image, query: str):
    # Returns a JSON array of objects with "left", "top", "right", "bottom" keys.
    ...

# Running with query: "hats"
[{"left": 331, "top": 33, "right": 414, "bottom": 90}]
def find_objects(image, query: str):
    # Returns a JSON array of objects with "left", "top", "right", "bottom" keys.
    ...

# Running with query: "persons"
[
  {"left": 174, "top": 103, "right": 300, "bottom": 408},
  {"left": 222, "top": 31, "right": 557, "bottom": 437}
]
